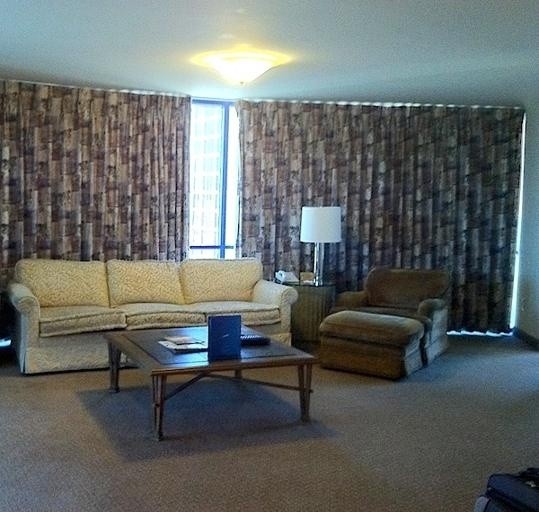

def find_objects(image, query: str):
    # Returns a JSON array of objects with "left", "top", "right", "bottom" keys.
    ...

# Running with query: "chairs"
[{"left": 329, "top": 267, "right": 450, "bottom": 367}]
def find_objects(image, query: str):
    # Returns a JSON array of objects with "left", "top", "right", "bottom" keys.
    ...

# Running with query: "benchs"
[{"left": 318, "top": 309, "right": 425, "bottom": 378}]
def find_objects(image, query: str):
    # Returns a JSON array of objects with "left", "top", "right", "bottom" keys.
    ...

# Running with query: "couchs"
[{"left": 7, "top": 269, "right": 299, "bottom": 376}]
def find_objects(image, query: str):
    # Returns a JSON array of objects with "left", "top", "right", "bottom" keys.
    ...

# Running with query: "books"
[{"left": 160, "top": 335, "right": 206, "bottom": 354}]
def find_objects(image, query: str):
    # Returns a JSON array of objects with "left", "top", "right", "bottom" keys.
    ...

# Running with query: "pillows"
[
  {"left": 180, "top": 257, "right": 263, "bottom": 304},
  {"left": 14, "top": 258, "right": 110, "bottom": 307},
  {"left": 107, "top": 258, "right": 183, "bottom": 307}
]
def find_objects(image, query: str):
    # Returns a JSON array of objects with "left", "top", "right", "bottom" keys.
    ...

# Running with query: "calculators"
[{"left": 240, "top": 333, "right": 270, "bottom": 346}]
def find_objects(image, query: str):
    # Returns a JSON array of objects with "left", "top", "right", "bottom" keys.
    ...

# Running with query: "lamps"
[{"left": 299, "top": 206, "right": 342, "bottom": 286}]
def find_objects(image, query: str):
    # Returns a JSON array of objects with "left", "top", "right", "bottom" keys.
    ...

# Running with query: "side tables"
[{"left": 273, "top": 277, "right": 336, "bottom": 342}]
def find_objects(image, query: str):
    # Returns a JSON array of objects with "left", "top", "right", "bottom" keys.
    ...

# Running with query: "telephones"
[{"left": 275, "top": 270, "right": 300, "bottom": 283}]
